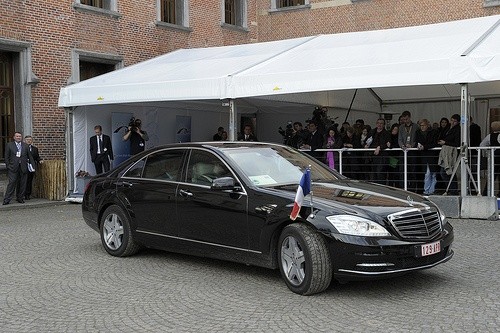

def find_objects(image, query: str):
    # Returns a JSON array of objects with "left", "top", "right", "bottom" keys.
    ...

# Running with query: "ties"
[
  {"left": 18, "top": 144, "right": 20, "bottom": 156},
  {"left": 99, "top": 137, "right": 104, "bottom": 155},
  {"left": 246, "top": 135, "right": 248, "bottom": 140}
]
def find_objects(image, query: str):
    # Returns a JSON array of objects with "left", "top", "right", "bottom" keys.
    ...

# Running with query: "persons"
[
  {"left": 468, "top": 116, "right": 482, "bottom": 196},
  {"left": 90, "top": 124, "right": 114, "bottom": 175},
  {"left": 479, "top": 120, "right": 500, "bottom": 197},
  {"left": 398, "top": 111, "right": 420, "bottom": 193},
  {"left": 3, "top": 132, "right": 36, "bottom": 205},
  {"left": 213, "top": 125, "right": 259, "bottom": 142},
  {"left": 14, "top": 135, "right": 44, "bottom": 203},
  {"left": 278, "top": 115, "right": 451, "bottom": 193},
  {"left": 437, "top": 114, "right": 461, "bottom": 195},
  {"left": 415, "top": 118, "right": 443, "bottom": 196},
  {"left": 122, "top": 119, "right": 150, "bottom": 159}
]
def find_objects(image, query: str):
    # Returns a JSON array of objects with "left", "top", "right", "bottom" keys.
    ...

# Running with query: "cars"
[{"left": 82, "top": 141, "right": 455, "bottom": 295}]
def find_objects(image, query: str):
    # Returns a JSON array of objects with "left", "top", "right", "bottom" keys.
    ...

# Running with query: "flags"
[{"left": 289, "top": 167, "right": 311, "bottom": 221}]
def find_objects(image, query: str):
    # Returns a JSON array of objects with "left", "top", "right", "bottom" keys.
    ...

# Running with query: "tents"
[{"left": 57, "top": 15, "right": 500, "bottom": 203}]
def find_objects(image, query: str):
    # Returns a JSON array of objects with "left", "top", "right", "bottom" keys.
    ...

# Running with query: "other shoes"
[
  {"left": 3, "top": 202, "right": 9, "bottom": 205},
  {"left": 18, "top": 200, "right": 24, "bottom": 202}
]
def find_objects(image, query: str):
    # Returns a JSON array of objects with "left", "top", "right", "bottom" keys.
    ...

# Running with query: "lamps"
[{"left": 24, "top": 70, "right": 40, "bottom": 87}]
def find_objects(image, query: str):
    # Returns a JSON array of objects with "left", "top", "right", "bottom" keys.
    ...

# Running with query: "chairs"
[
  {"left": 191, "top": 161, "right": 214, "bottom": 182},
  {"left": 144, "top": 160, "right": 168, "bottom": 180}
]
